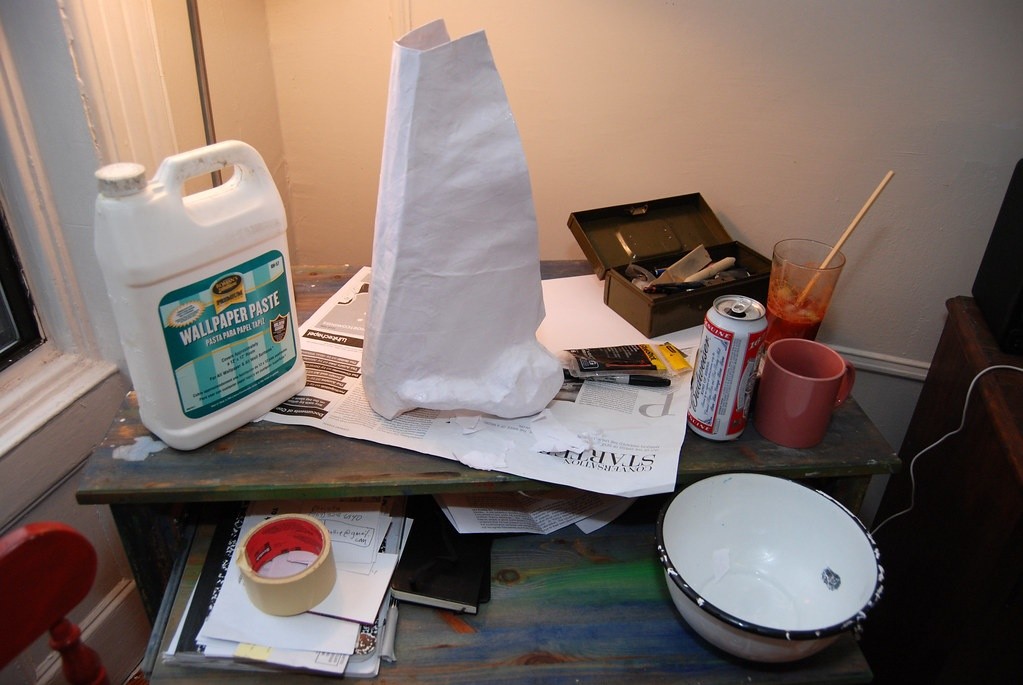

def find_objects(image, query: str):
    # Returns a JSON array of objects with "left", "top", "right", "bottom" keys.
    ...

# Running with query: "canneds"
[{"left": 688, "top": 294, "right": 767, "bottom": 441}]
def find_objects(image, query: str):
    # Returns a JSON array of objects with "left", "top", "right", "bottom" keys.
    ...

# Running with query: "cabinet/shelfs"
[{"left": 857, "top": 296, "right": 1023, "bottom": 685}]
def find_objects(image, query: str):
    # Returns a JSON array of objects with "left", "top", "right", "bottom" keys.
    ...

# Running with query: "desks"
[{"left": 74, "top": 260, "right": 903, "bottom": 685}]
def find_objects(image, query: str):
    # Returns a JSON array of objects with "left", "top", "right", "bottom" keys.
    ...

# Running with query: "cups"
[
  {"left": 763, "top": 238, "right": 845, "bottom": 350},
  {"left": 752, "top": 338, "right": 856, "bottom": 449}
]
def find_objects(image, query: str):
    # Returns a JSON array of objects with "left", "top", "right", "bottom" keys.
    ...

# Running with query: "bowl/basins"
[{"left": 657, "top": 470, "right": 884, "bottom": 663}]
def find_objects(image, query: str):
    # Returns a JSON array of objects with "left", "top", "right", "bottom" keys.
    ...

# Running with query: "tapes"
[{"left": 235, "top": 513, "right": 337, "bottom": 616}]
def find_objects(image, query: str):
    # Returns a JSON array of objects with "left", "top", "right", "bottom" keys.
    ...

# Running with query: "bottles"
[{"left": 94, "top": 139, "right": 306, "bottom": 451}]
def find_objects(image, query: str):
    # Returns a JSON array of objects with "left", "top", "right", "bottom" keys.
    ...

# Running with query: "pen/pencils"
[
  {"left": 643, "top": 285, "right": 682, "bottom": 294},
  {"left": 564, "top": 368, "right": 671, "bottom": 387}
]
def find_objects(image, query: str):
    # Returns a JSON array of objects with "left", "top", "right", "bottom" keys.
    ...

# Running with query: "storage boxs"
[{"left": 566, "top": 192, "right": 773, "bottom": 340}]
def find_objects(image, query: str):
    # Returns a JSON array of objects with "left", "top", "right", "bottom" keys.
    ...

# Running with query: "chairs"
[{"left": 0, "top": 520, "right": 110, "bottom": 685}]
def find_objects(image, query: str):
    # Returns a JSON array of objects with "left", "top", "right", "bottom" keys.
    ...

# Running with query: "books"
[{"left": 163, "top": 494, "right": 495, "bottom": 678}]
